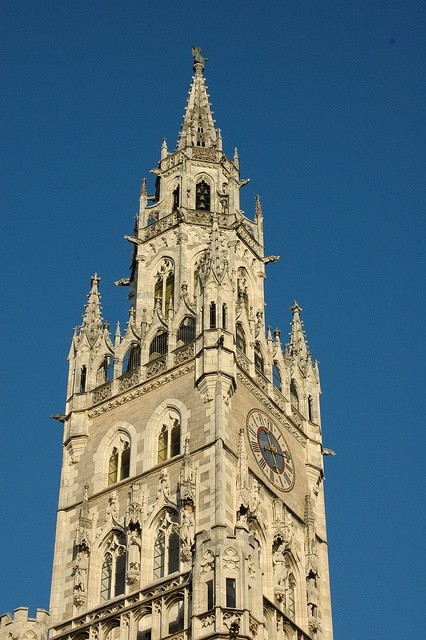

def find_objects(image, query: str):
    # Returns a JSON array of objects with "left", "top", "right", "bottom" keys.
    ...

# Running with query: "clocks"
[{"left": 245, "top": 409, "right": 295, "bottom": 493}]
